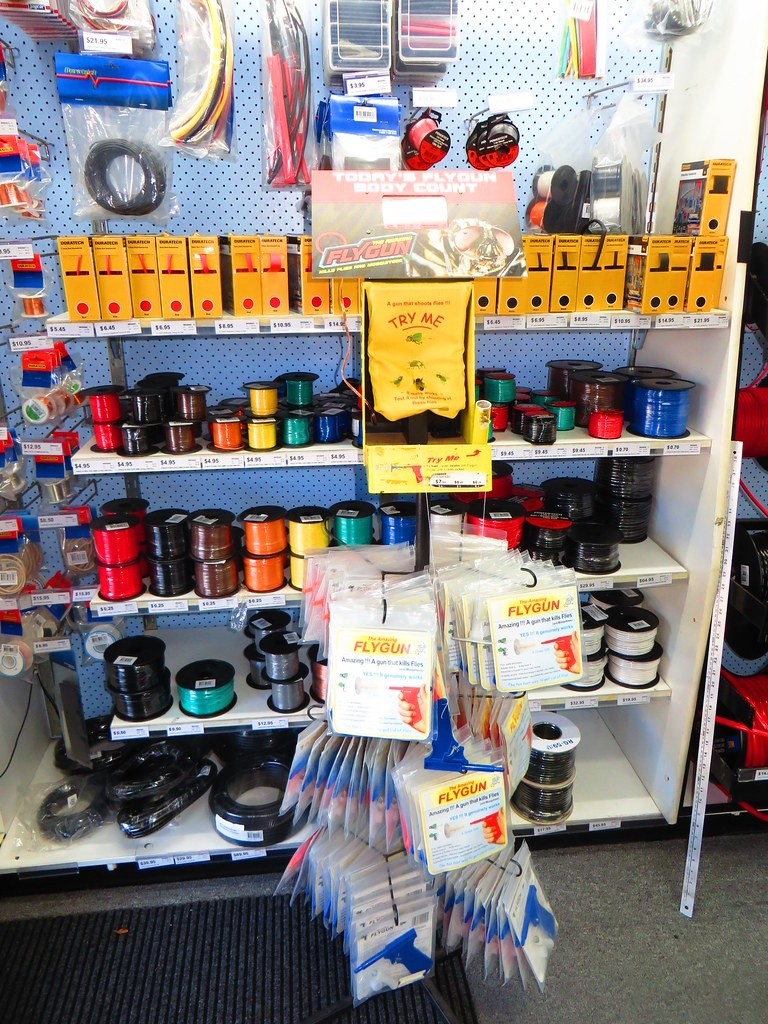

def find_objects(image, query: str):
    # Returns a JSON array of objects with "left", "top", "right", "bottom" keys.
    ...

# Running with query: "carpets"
[{"left": 0, "top": 892, "right": 478, "bottom": 1024}]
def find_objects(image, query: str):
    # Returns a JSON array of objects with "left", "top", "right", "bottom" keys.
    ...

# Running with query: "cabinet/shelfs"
[{"left": 0, "top": 309, "right": 731, "bottom": 901}]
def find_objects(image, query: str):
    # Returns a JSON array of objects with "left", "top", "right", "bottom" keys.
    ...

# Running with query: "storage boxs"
[
  {"left": 56, "top": 156, "right": 738, "bottom": 320},
  {"left": 322, "top": 0, "right": 459, "bottom": 89}
]
